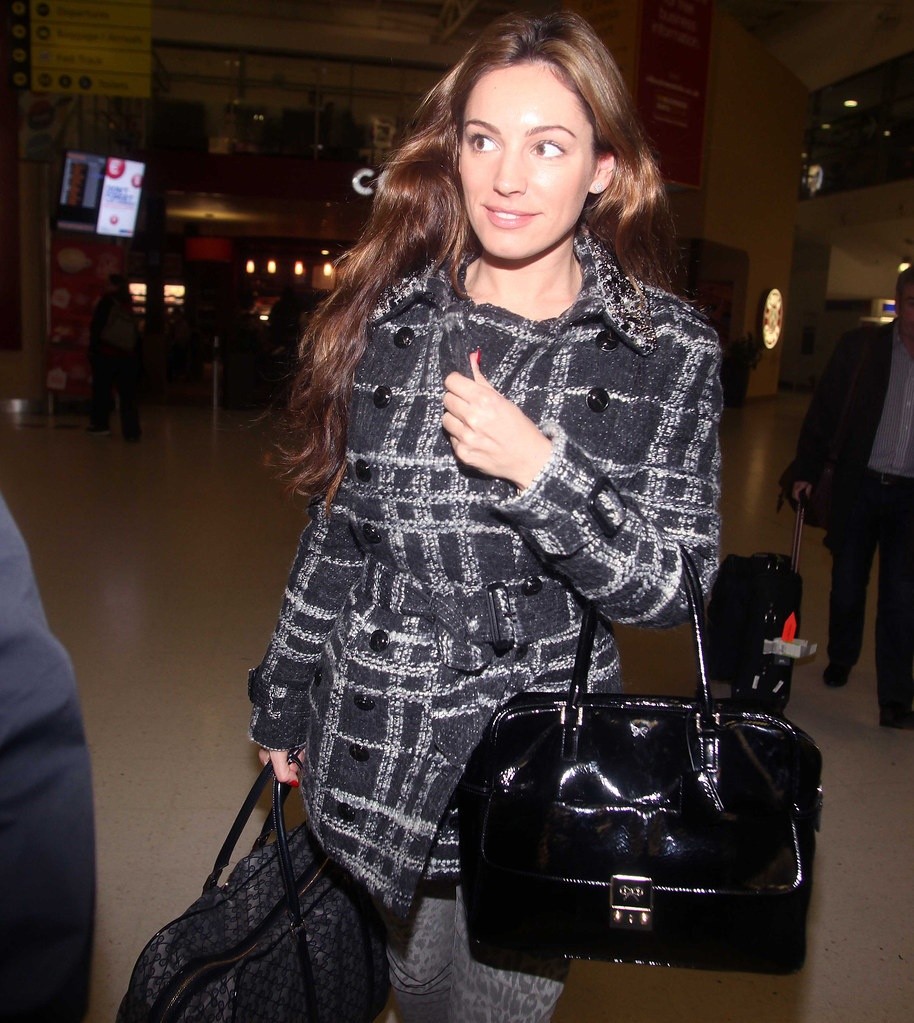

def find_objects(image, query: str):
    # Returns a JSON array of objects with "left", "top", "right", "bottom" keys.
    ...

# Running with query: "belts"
[{"left": 865, "top": 468, "right": 914, "bottom": 487}]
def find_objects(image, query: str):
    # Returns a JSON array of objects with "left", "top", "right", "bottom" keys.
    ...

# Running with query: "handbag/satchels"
[
  {"left": 115, "top": 747, "right": 390, "bottom": 1023},
  {"left": 454, "top": 547, "right": 823, "bottom": 976}
]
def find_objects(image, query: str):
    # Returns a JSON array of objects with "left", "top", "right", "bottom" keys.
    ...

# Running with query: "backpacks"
[{"left": 102, "top": 295, "right": 139, "bottom": 352}]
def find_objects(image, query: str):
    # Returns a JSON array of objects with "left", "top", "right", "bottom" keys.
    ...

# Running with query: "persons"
[
  {"left": 0, "top": 485, "right": 97, "bottom": 1023},
  {"left": 267, "top": 285, "right": 309, "bottom": 338},
  {"left": 789, "top": 265, "right": 914, "bottom": 729},
  {"left": 86, "top": 273, "right": 142, "bottom": 441},
  {"left": 248, "top": 14, "right": 720, "bottom": 1023},
  {"left": 307, "top": 91, "right": 322, "bottom": 110}
]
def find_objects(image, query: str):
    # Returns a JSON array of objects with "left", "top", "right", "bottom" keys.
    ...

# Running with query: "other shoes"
[
  {"left": 822, "top": 658, "right": 852, "bottom": 687},
  {"left": 85, "top": 425, "right": 112, "bottom": 435},
  {"left": 880, "top": 707, "right": 914, "bottom": 730},
  {"left": 125, "top": 434, "right": 140, "bottom": 442}
]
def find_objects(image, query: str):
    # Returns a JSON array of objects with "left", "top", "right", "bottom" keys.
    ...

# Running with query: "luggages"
[{"left": 706, "top": 489, "right": 807, "bottom": 712}]
